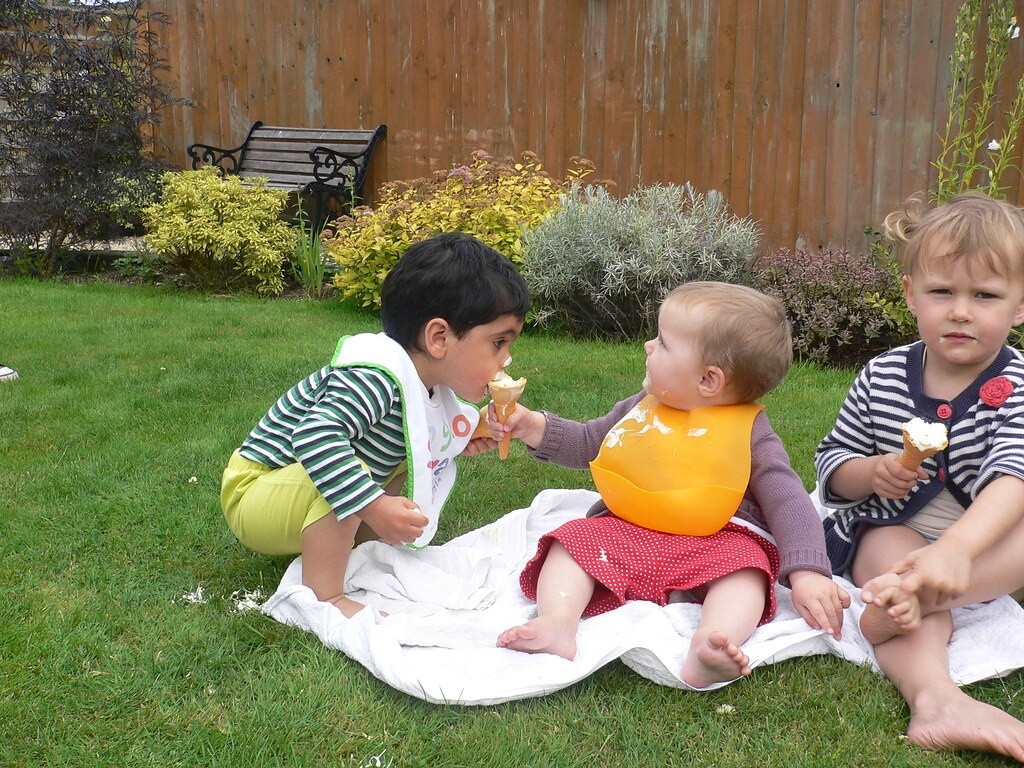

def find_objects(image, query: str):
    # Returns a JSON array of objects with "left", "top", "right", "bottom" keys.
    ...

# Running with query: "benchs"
[{"left": 187, "top": 121, "right": 388, "bottom": 250}]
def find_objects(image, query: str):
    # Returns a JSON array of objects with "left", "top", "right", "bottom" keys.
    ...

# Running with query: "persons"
[
  {"left": 490, "top": 281, "right": 852, "bottom": 690},
  {"left": 815, "top": 189, "right": 1023, "bottom": 763},
  {"left": 219, "top": 229, "right": 498, "bottom": 620}
]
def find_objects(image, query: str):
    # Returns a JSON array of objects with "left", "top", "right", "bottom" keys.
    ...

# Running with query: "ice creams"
[
  {"left": 488, "top": 371, "right": 526, "bottom": 461},
  {"left": 899, "top": 415, "right": 949, "bottom": 471}
]
